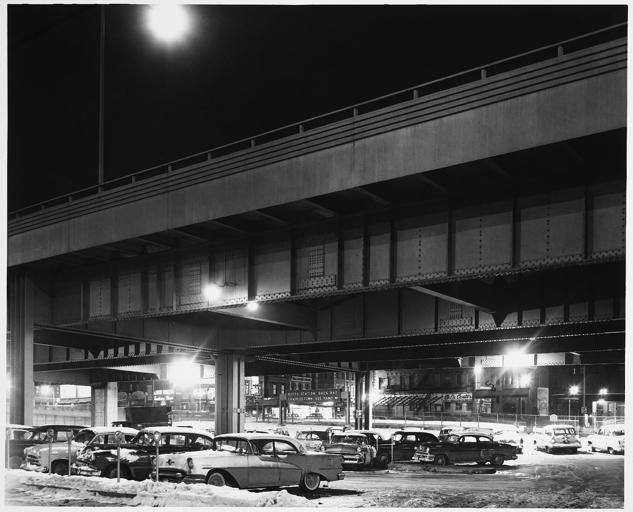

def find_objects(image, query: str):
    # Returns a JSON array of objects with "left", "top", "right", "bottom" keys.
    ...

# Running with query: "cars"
[
  {"left": 587, "top": 423, "right": 625, "bottom": 456},
  {"left": 532, "top": 421, "right": 583, "bottom": 454}
]
